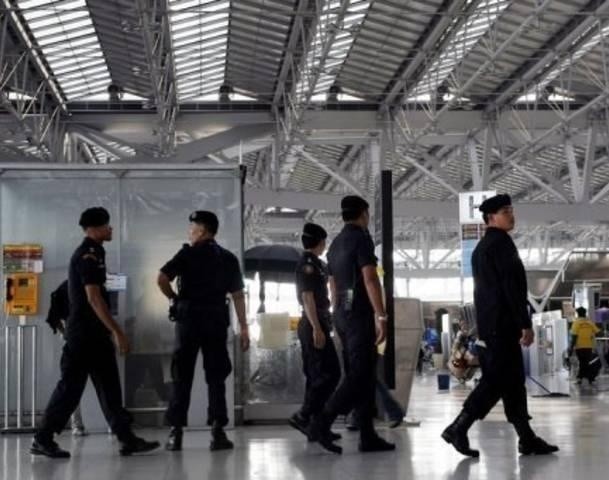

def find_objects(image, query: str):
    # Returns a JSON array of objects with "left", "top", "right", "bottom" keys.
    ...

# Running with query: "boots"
[
  {"left": 290, "top": 402, "right": 344, "bottom": 457},
  {"left": 113, "top": 426, "right": 162, "bottom": 458},
  {"left": 514, "top": 420, "right": 560, "bottom": 458},
  {"left": 164, "top": 427, "right": 185, "bottom": 452},
  {"left": 29, "top": 422, "right": 71, "bottom": 461},
  {"left": 357, "top": 421, "right": 397, "bottom": 453},
  {"left": 442, "top": 409, "right": 481, "bottom": 460},
  {"left": 207, "top": 419, "right": 235, "bottom": 453}
]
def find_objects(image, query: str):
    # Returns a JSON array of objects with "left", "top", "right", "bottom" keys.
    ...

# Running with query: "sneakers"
[{"left": 73, "top": 425, "right": 87, "bottom": 439}]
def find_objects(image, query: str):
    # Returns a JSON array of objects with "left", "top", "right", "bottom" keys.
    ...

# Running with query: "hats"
[
  {"left": 188, "top": 210, "right": 218, "bottom": 223},
  {"left": 340, "top": 195, "right": 371, "bottom": 213},
  {"left": 78, "top": 206, "right": 111, "bottom": 228},
  {"left": 302, "top": 223, "right": 327, "bottom": 239},
  {"left": 479, "top": 193, "right": 515, "bottom": 213}
]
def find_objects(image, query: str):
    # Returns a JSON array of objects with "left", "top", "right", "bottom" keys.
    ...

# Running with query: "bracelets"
[{"left": 371, "top": 312, "right": 388, "bottom": 321}]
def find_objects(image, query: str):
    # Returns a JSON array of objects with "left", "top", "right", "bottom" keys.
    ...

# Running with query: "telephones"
[{"left": 5, "top": 278, "right": 13, "bottom": 302}]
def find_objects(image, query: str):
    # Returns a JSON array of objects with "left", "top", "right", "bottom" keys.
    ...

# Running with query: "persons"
[
  {"left": 450, "top": 317, "right": 474, "bottom": 353},
  {"left": 306, "top": 193, "right": 398, "bottom": 455},
  {"left": 339, "top": 325, "right": 408, "bottom": 433},
  {"left": 595, "top": 303, "right": 609, "bottom": 328},
  {"left": 440, "top": 191, "right": 562, "bottom": 459},
  {"left": 566, "top": 306, "right": 601, "bottom": 382},
  {"left": 28, "top": 205, "right": 161, "bottom": 461},
  {"left": 288, "top": 223, "right": 347, "bottom": 441},
  {"left": 157, "top": 208, "right": 252, "bottom": 453}
]
